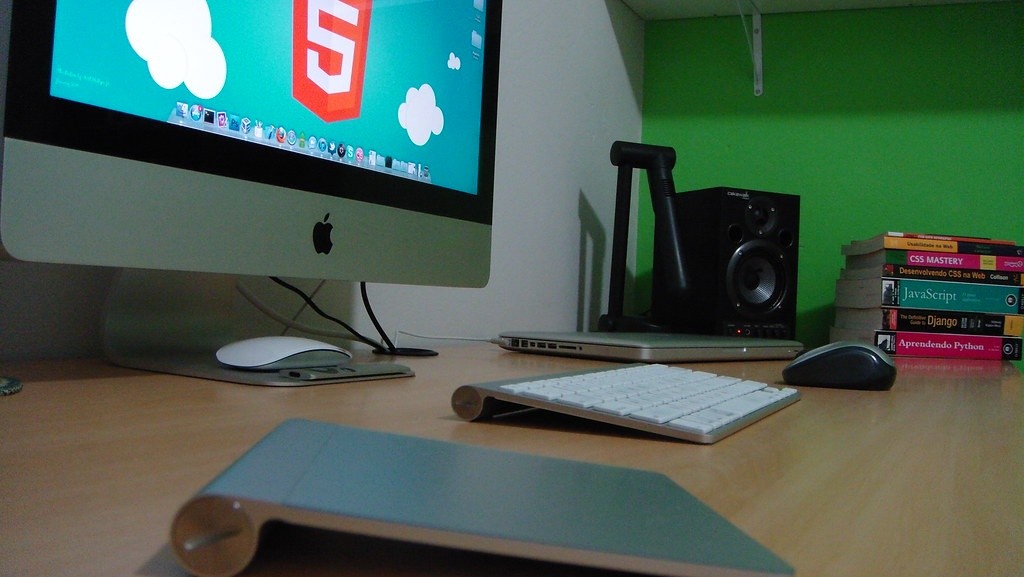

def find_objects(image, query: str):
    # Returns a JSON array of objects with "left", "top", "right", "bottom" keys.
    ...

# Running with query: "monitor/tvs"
[{"left": 0, "top": 0, "right": 505, "bottom": 388}]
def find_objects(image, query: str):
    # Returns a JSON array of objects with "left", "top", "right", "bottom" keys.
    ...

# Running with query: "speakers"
[{"left": 651, "top": 187, "right": 799, "bottom": 341}]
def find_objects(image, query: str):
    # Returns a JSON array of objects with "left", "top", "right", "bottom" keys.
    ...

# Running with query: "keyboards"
[{"left": 451, "top": 363, "right": 798, "bottom": 445}]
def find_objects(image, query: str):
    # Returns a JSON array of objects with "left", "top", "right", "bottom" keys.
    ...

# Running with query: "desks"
[{"left": 0, "top": 340, "right": 1024, "bottom": 577}]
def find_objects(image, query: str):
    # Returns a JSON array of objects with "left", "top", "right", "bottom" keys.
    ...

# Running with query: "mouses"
[
  {"left": 216, "top": 336, "right": 352, "bottom": 371},
  {"left": 781, "top": 341, "right": 897, "bottom": 391}
]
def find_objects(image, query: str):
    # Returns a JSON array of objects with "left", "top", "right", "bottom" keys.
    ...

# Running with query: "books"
[
  {"left": 827, "top": 326, "right": 1023, "bottom": 365},
  {"left": 828, "top": 232, "right": 1024, "bottom": 336}
]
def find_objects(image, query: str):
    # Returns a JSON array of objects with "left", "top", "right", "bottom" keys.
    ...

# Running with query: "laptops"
[{"left": 500, "top": 331, "right": 804, "bottom": 362}]
